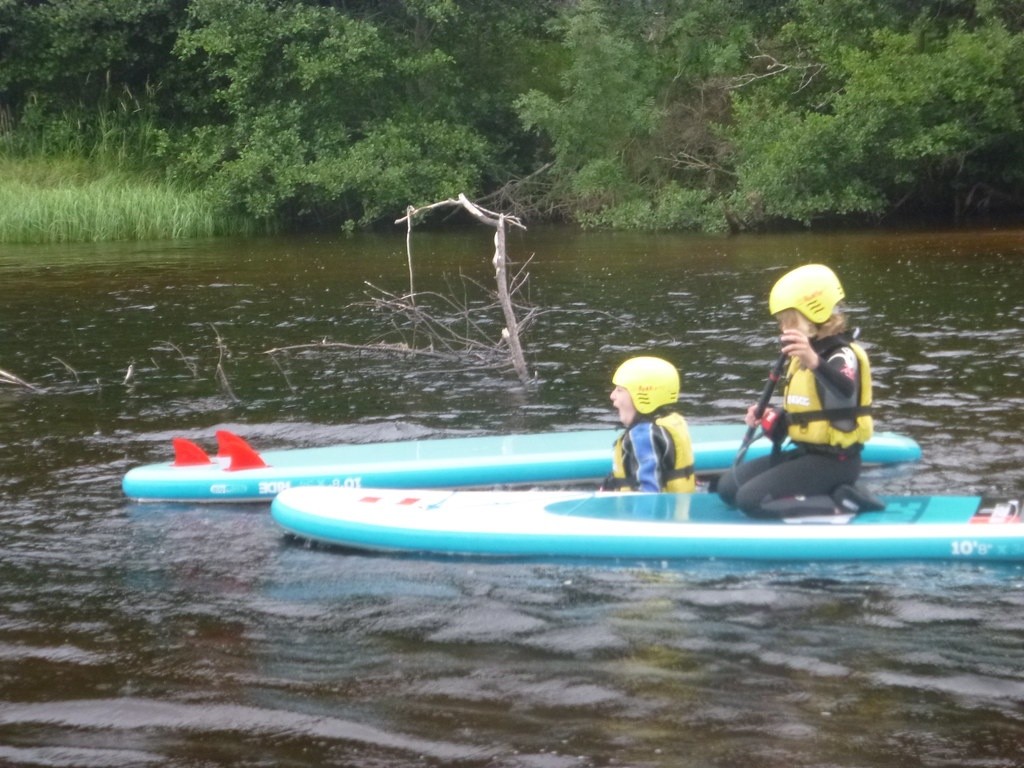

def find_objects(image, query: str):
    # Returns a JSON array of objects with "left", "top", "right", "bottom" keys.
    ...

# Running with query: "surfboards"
[
  {"left": 122, "top": 425, "right": 922, "bottom": 504},
  {"left": 271, "top": 484, "right": 1024, "bottom": 562}
]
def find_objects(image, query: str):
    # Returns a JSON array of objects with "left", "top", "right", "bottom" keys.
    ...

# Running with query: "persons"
[
  {"left": 598, "top": 356, "right": 697, "bottom": 521},
  {"left": 718, "top": 264, "right": 886, "bottom": 521}
]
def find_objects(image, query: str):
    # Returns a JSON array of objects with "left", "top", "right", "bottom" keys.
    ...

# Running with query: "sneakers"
[{"left": 831, "top": 484, "right": 885, "bottom": 512}]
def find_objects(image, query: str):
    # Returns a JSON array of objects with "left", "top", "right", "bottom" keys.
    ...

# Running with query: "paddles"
[{"left": 735, "top": 341, "right": 794, "bottom": 468}]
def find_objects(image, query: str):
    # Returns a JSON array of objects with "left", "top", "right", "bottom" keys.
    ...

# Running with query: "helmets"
[
  {"left": 612, "top": 356, "right": 680, "bottom": 414},
  {"left": 769, "top": 263, "right": 846, "bottom": 323}
]
